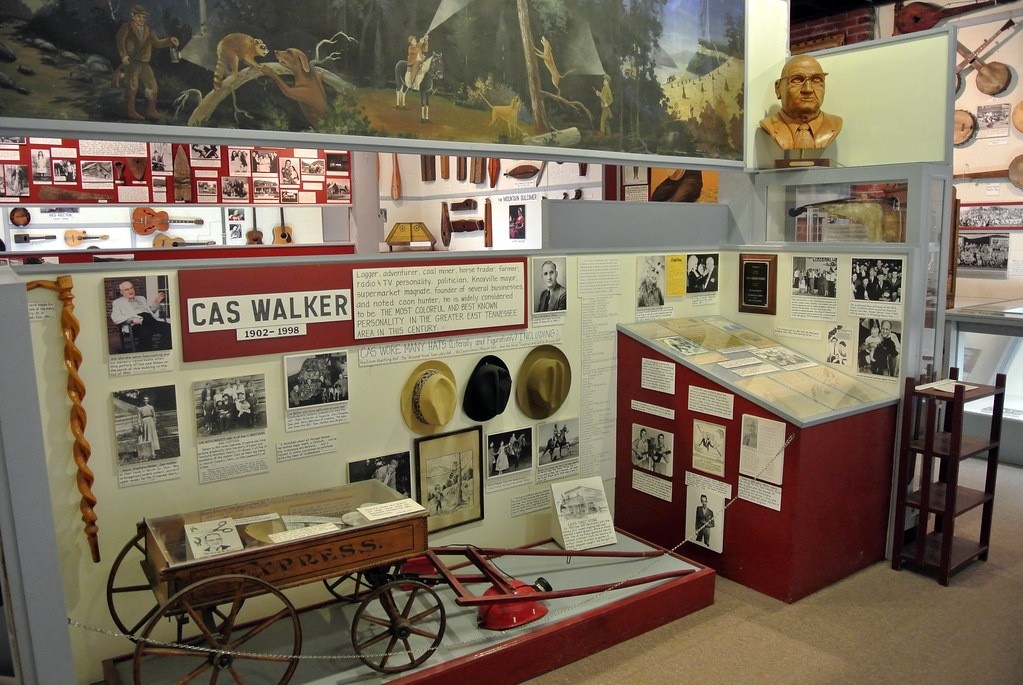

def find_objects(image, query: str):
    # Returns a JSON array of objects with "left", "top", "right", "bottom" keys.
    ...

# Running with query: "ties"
[{"left": 543, "top": 292, "right": 550, "bottom": 311}]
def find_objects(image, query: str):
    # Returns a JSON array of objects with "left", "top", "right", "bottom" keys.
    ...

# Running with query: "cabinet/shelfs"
[{"left": 892, "top": 365, "right": 1008, "bottom": 586}]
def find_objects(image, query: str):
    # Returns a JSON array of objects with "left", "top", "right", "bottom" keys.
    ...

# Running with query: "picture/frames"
[
  {"left": 0, "top": 0, "right": 751, "bottom": 171},
  {"left": 414, "top": 424, "right": 485, "bottom": 536},
  {"left": 346, "top": 451, "right": 413, "bottom": 499},
  {"left": 738, "top": 253, "right": 778, "bottom": 315}
]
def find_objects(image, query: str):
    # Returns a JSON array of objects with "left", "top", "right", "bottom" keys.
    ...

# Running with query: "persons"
[
  {"left": 434, "top": 487, "right": 444, "bottom": 515},
  {"left": 859, "top": 320, "right": 901, "bottom": 377},
  {"left": 827, "top": 337, "right": 848, "bottom": 365},
  {"left": 760, "top": 55, "right": 843, "bottom": 151},
  {"left": 372, "top": 460, "right": 398, "bottom": 490},
  {"left": 11, "top": 166, "right": 25, "bottom": 191},
  {"left": 794, "top": 262, "right": 836, "bottom": 296},
  {"left": 35, "top": 151, "right": 47, "bottom": 177},
  {"left": 687, "top": 256, "right": 717, "bottom": 291},
  {"left": 636, "top": 256, "right": 664, "bottom": 307},
  {"left": 198, "top": 379, "right": 256, "bottom": 433},
  {"left": 289, "top": 356, "right": 347, "bottom": 406},
  {"left": 957, "top": 242, "right": 1009, "bottom": 268},
  {"left": 64, "top": 161, "right": 75, "bottom": 181},
  {"left": 694, "top": 494, "right": 716, "bottom": 547},
  {"left": 536, "top": 261, "right": 567, "bottom": 312},
  {"left": 515, "top": 208, "right": 525, "bottom": 231},
  {"left": 111, "top": 281, "right": 172, "bottom": 351},
  {"left": 553, "top": 424, "right": 561, "bottom": 447},
  {"left": 488, "top": 433, "right": 518, "bottom": 476},
  {"left": 151, "top": 144, "right": 172, "bottom": 171},
  {"left": 960, "top": 207, "right": 1023, "bottom": 226},
  {"left": 852, "top": 259, "right": 902, "bottom": 302},
  {"left": 223, "top": 150, "right": 322, "bottom": 198},
  {"left": 137, "top": 395, "right": 161, "bottom": 451},
  {"left": 632, "top": 429, "right": 670, "bottom": 475},
  {"left": 743, "top": 418, "right": 757, "bottom": 448}
]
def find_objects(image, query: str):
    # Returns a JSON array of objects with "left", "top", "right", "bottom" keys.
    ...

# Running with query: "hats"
[
  {"left": 516, "top": 344, "right": 572, "bottom": 420},
  {"left": 237, "top": 392, "right": 244, "bottom": 395},
  {"left": 464, "top": 356, "right": 512, "bottom": 421},
  {"left": 400, "top": 360, "right": 457, "bottom": 437},
  {"left": 222, "top": 394, "right": 229, "bottom": 397}
]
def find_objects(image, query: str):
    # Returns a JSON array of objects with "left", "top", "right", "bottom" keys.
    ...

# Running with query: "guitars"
[
  {"left": 152, "top": 232, "right": 216, "bottom": 247},
  {"left": 132, "top": 208, "right": 204, "bottom": 235},
  {"left": 244, "top": 207, "right": 264, "bottom": 245},
  {"left": 65, "top": 229, "right": 110, "bottom": 247},
  {"left": 271, "top": 207, "right": 294, "bottom": 244},
  {"left": 632, "top": 446, "right": 662, "bottom": 464}
]
